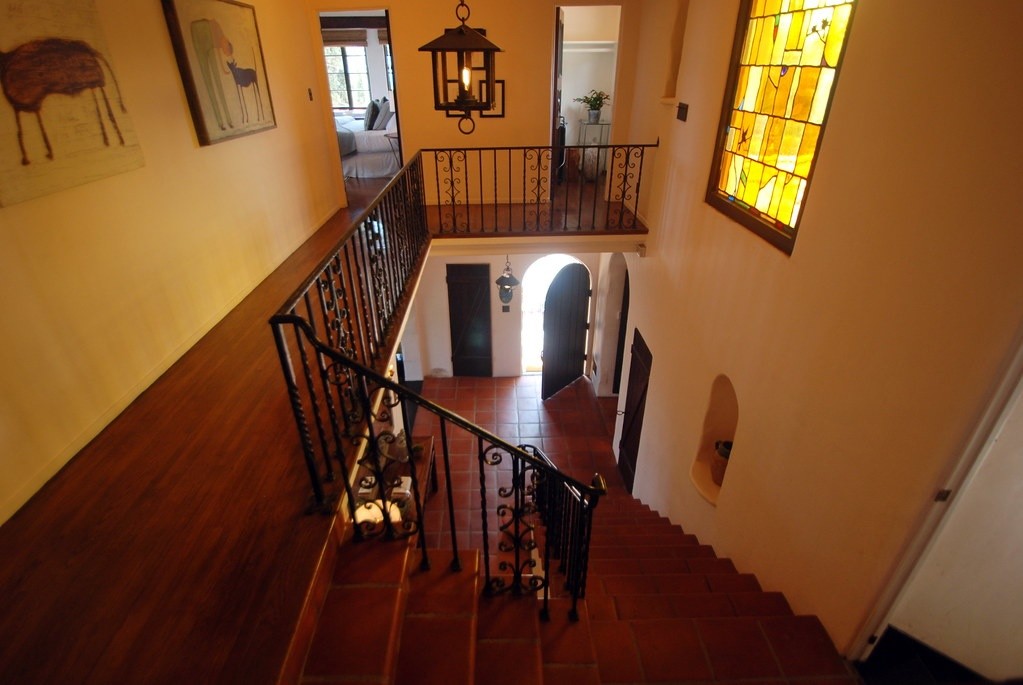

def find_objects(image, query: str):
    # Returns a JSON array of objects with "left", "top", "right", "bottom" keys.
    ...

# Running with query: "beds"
[{"left": 334, "top": 113, "right": 401, "bottom": 179}]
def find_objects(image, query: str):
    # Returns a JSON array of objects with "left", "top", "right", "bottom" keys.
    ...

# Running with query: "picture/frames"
[{"left": 160, "top": 0, "right": 278, "bottom": 147}]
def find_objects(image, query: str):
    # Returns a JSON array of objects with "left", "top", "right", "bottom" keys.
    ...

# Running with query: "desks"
[
  {"left": 577, "top": 120, "right": 612, "bottom": 186},
  {"left": 352, "top": 435, "right": 438, "bottom": 523}
]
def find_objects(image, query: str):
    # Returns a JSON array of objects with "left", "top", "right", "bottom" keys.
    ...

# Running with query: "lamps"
[
  {"left": 495, "top": 254, "right": 519, "bottom": 303},
  {"left": 417, "top": 1, "right": 504, "bottom": 135}
]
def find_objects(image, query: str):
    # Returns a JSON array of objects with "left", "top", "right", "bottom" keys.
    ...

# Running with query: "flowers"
[{"left": 572, "top": 89, "right": 611, "bottom": 110}]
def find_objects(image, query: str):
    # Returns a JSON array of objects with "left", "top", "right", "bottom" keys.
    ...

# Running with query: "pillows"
[{"left": 364, "top": 97, "right": 394, "bottom": 131}]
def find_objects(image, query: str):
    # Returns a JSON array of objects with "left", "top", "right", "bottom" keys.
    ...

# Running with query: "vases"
[{"left": 588, "top": 109, "right": 601, "bottom": 123}]
